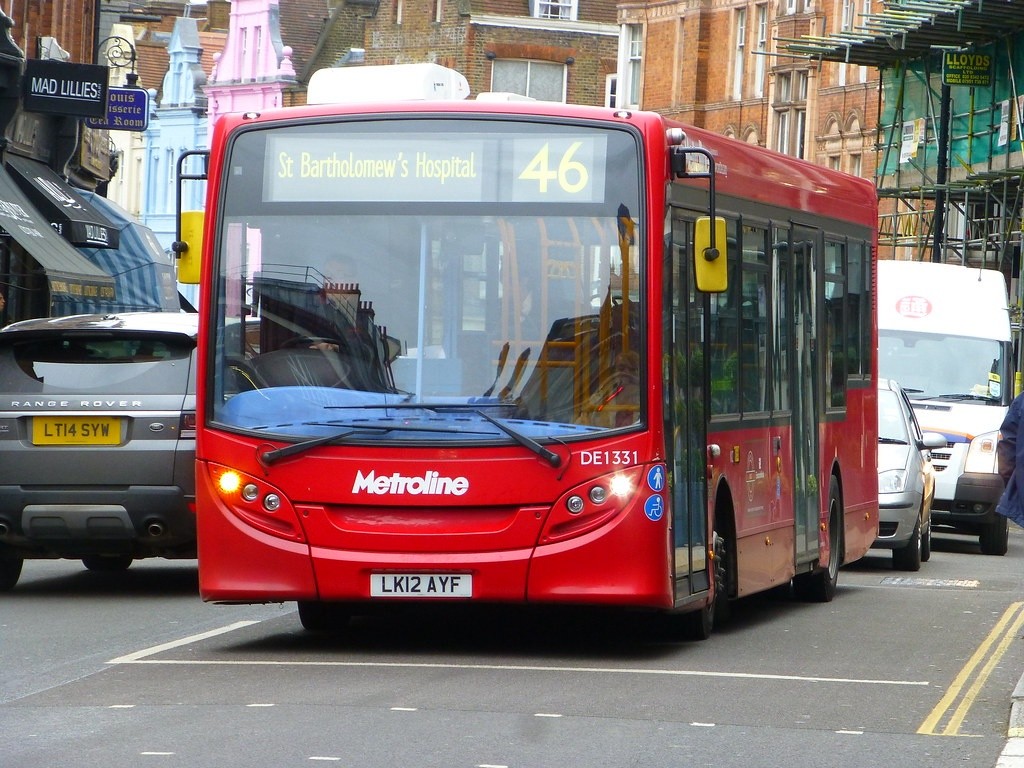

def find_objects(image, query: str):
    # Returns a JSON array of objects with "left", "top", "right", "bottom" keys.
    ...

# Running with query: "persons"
[
  {"left": 995, "top": 391, "right": 1023, "bottom": 533},
  {"left": 273, "top": 254, "right": 372, "bottom": 358},
  {"left": 484, "top": 242, "right": 558, "bottom": 364}
]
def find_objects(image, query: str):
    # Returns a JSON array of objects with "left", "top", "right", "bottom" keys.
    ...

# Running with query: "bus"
[{"left": 172, "top": 64, "right": 879, "bottom": 640}]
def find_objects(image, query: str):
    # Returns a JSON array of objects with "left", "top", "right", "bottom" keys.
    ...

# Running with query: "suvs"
[{"left": 1, "top": 313, "right": 260, "bottom": 594}]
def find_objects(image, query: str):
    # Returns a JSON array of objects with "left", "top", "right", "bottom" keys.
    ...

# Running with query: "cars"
[{"left": 871, "top": 378, "right": 946, "bottom": 572}]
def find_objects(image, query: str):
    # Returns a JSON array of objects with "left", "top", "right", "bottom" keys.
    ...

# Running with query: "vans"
[{"left": 876, "top": 260, "right": 1015, "bottom": 555}]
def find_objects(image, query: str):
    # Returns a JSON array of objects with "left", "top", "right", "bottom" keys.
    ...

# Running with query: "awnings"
[{"left": 0, "top": 149, "right": 182, "bottom": 318}]
